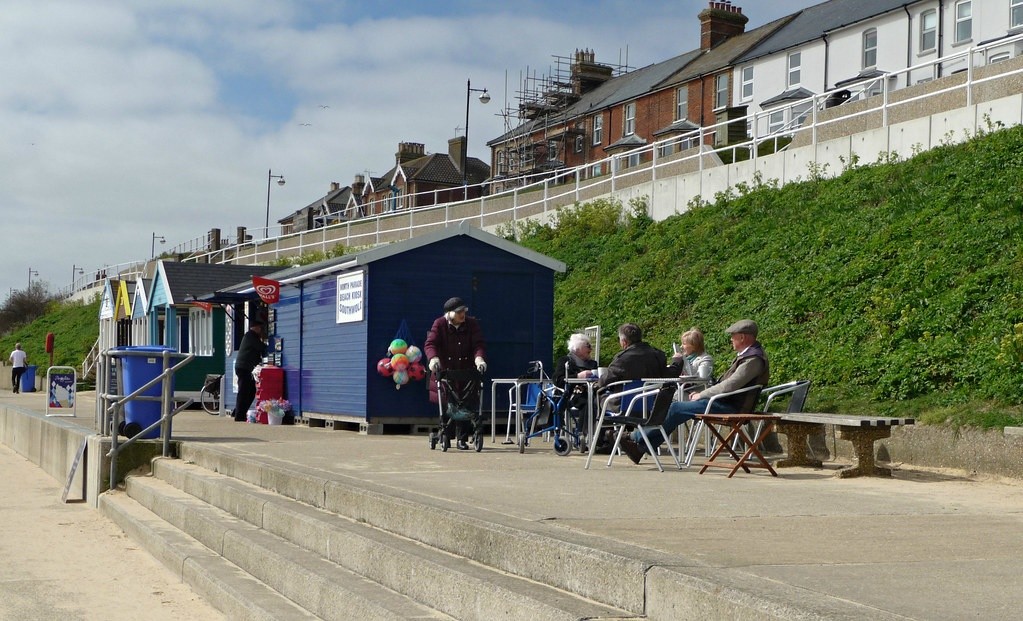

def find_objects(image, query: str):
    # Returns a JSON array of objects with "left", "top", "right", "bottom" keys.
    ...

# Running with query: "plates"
[{"left": 679, "top": 375, "right": 698, "bottom": 379}]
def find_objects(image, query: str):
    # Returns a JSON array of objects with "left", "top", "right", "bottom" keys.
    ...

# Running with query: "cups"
[
  {"left": 591, "top": 369, "right": 598, "bottom": 376},
  {"left": 598, "top": 367, "right": 608, "bottom": 378}
]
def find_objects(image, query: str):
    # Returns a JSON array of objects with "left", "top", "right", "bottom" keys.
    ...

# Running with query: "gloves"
[
  {"left": 475, "top": 356, "right": 487, "bottom": 372},
  {"left": 429, "top": 356, "right": 440, "bottom": 373}
]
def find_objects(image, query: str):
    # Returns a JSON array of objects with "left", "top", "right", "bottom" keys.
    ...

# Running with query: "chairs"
[{"left": 504, "top": 380, "right": 813, "bottom": 473}]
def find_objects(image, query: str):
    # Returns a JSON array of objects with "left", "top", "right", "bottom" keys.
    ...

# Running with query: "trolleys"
[
  {"left": 429, "top": 364, "right": 484, "bottom": 452},
  {"left": 516, "top": 360, "right": 587, "bottom": 456}
]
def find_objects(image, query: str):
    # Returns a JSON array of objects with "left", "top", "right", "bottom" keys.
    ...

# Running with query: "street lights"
[
  {"left": 72, "top": 267, "right": 85, "bottom": 292},
  {"left": 10, "top": 289, "right": 17, "bottom": 304},
  {"left": 29, "top": 271, "right": 39, "bottom": 301},
  {"left": 266, "top": 174, "right": 286, "bottom": 239},
  {"left": 461, "top": 89, "right": 491, "bottom": 201},
  {"left": 152, "top": 236, "right": 166, "bottom": 260}
]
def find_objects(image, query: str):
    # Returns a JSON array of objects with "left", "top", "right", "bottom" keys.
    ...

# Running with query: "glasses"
[{"left": 584, "top": 344, "right": 591, "bottom": 349}]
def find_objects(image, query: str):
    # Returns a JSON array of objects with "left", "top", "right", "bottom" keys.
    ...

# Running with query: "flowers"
[{"left": 259, "top": 398, "right": 292, "bottom": 413}]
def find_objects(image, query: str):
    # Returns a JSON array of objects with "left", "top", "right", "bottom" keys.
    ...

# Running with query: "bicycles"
[{"left": 201, "top": 374, "right": 220, "bottom": 415}]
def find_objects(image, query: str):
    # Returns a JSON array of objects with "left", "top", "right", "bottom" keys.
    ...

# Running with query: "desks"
[
  {"left": 642, "top": 377, "right": 712, "bottom": 465},
  {"left": 562, "top": 378, "right": 608, "bottom": 455},
  {"left": 490, "top": 377, "right": 553, "bottom": 442},
  {"left": 694, "top": 412, "right": 784, "bottom": 477}
]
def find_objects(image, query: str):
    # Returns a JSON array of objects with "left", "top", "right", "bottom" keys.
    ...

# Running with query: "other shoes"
[
  {"left": 13, "top": 386, "right": 19, "bottom": 394},
  {"left": 596, "top": 440, "right": 603, "bottom": 447},
  {"left": 575, "top": 444, "right": 588, "bottom": 451}
]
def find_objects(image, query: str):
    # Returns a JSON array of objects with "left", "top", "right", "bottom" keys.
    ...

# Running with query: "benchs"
[{"left": 773, "top": 411, "right": 916, "bottom": 478}]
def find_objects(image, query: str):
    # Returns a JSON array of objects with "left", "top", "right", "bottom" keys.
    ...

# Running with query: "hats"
[
  {"left": 726, "top": 319, "right": 758, "bottom": 334},
  {"left": 444, "top": 297, "right": 465, "bottom": 311},
  {"left": 250, "top": 320, "right": 264, "bottom": 327}
]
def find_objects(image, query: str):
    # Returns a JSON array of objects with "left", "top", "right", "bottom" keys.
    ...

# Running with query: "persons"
[
  {"left": 672, "top": 327, "right": 712, "bottom": 402},
  {"left": 593, "top": 323, "right": 667, "bottom": 455},
  {"left": 422, "top": 296, "right": 488, "bottom": 450},
  {"left": 554, "top": 333, "right": 603, "bottom": 445},
  {"left": 606, "top": 319, "right": 769, "bottom": 463},
  {"left": 9, "top": 343, "right": 27, "bottom": 393},
  {"left": 231, "top": 319, "right": 270, "bottom": 422}
]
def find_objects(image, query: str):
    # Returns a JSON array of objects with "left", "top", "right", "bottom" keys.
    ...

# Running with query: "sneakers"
[{"left": 606, "top": 429, "right": 645, "bottom": 464}]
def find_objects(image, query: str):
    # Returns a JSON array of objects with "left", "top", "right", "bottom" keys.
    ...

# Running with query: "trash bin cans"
[
  {"left": 21, "top": 365, "right": 37, "bottom": 392},
  {"left": 111, "top": 346, "right": 177, "bottom": 439}
]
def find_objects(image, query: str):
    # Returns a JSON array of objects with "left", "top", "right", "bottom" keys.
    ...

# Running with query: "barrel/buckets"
[{"left": 268, "top": 409, "right": 285, "bottom": 425}]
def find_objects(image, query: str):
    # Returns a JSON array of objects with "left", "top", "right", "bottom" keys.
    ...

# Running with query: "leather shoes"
[
  {"left": 457, "top": 440, "right": 469, "bottom": 449},
  {"left": 447, "top": 440, "right": 451, "bottom": 448}
]
